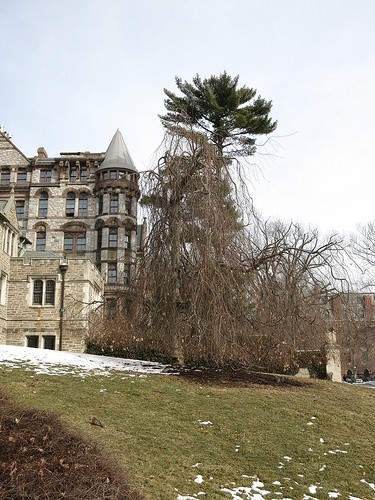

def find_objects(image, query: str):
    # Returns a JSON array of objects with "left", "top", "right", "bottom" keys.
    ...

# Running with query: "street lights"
[{"left": 58, "top": 263, "right": 68, "bottom": 351}]
